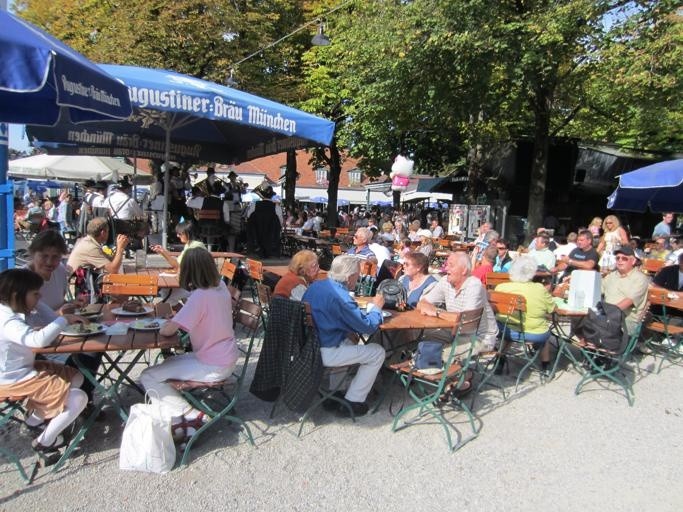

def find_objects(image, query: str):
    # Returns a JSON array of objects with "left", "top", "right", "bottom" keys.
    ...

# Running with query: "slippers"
[
  {"left": 440, "top": 381, "right": 472, "bottom": 401},
  {"left": 448, "top": 370, "right": 473, "bottom": 381}
]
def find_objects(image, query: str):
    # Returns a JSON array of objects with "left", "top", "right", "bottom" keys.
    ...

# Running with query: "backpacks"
[
  {"left": 376, "top": 277, "right": 408, "bottom": 312},
  {"left": 575, "top": 300, "right": 628, "bottom": 353}
]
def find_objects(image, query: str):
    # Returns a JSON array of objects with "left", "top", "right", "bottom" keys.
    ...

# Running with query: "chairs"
[
  {"left": 486, "top": 271, "right": 510, "bottom": 286},
  {"left": 287, "top": 227, "right": 349, "bottom": 255},
  {"left": 577, "top": 302, "right": 651, "bottom": 406},
  {"left": 493, "top": 290, "right": 547, "bottom": 393},
  {"left": 160, "top": 298, "right": 264, "bottom": 469},
  {"left": 648, "top": 288, "right": 683, "bottom": 375},
  {"left": 258, "top": 284, "right": 273, "bottom": 309},
  {"left": 387, "top": 308, "right": 482, "bottom": 452},
  {"left": 198, "top": 209, "right": 223, "bottom": 251},
  {"left": 645, "top": 258, "right": 664, "bottom": 272},
  {"left": 100, "top": 273, "right": 159, "bottom": 306},
  {"left": 0, "top": 391, "right": 38, "bottom": 484},
  {"left": 245, "top": 259, "right": 263, "bottom": 284},
  {"left": 219, "top": 260, "right": 237, "bottom": 280},
  {"left": 434, "top": 235, "right": 467, "bottom": 255},
  {"left": 268, "top": 298, "right": 359, "bottom": 438},
  {"left": 474, "top": 294, "right": 519, "bottom": 408}
]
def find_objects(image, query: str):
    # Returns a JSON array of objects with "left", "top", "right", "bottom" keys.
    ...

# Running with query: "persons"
[
  {"left": 150, "top": 172, "right": 164, "bottom": 198},
  {"left": 550, "top": 230, "right": 598, "bottom": 280},
  {"left": 66, "top": 215, "right": 130, "bottom": 281},
  {"left": 271, "top": 249, "right": 320, "bottom": 302},
  {"left": 300, "top": 255, "right": 386, "bottom": 416},
  {"left": 250, "top": 187, "right": 285, "bottom": 233},
  {"left": 397, "top": 251, "right": 435, "bottom": 309},
  {"left": 194, "top": 167, "right": 234, "bottom": 249},
  {"left": 588, "top": 216, "right": 602, "bottom": 247},
  {"left": 495, "top": 272, "right": 549, "bottom": 376},
  {"left": 170, "top": 165, "right": 185, "bottom": 216},
  {"left": 74, "top": 180, "right": 96, "bottom": 215},
  {"left": 530, "top": 236, "right": 555, "bottom": 268},
  {"left": 556, "top": 232, "right": 577, "bottom": 256},
  {"left": 19, "top": 230, "right": 109, "bottom": 423},
  {"left": 476, "top": 231, "right": 500, "bottom": 252},
  {"left": 82, "top": 180, "right": 106, "bottom": 211},
  {"left": 417, "top": 252, "right": 498, "bottom": 404},
  {"left": 472, "top": 248, "right": 496, "bottom": 284},
  {"left": 346, "top": 227, "right": 377, "bottom": 257},
  {"left": 0, "top": 270, "right": 90, "bottom": 450},
  {"left": 228, "top": 172, "right": 248, "bottom": 251},
  {"left": 598, "top": 214, "right": 629, "bottom": 273},
  {"left": 15, "top": 186, "right": 73, "bottom": 236},
  {"left": 599, "top": 247, "right": 649, "bottom": 366},
  {"left": 101, "top": 177, "right": 145, "bottom": 234},
  {"left": 653, "top": 253, "right": 683, "bottom": 290},
  {"left": 148, "top": 219, "right": 206, "bottom": 270},
  {"left": 287, "top": 198, "right": 446, "bottom": 237},
  {"left": 652, "top": 213, "right": 673, "bottom": 241},
  {"left": 140, "top": 247, "right": 239, "bottom": 454},
  {"left": 368, "top": 228, "right": 393, "bottom": 266},
  {"left": 494, "top": 240, "right": 514, "bottom": 263}
]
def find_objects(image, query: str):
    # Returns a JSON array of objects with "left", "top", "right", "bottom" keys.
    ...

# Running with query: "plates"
[
  {"left": 360, "top": 309, "right": 391, "bottom": 317},
  {"left": 59, "top": 322, "right": 108, "bottom": 337},
  {"left": 110, "top": 306, "right": 154, "bottom": 316},
  {"left": 128, "top": 318, "right": 170, "bottom": 331}
]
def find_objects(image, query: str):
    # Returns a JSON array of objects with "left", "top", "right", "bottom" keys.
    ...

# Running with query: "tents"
[{"left": 571, "top": 143, "right": 679, "bottom": 187}]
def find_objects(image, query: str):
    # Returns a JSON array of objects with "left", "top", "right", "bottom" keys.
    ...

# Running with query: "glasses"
[
  {"left": 355, "top": 235, "right": 362, "bottom": 239},
  {"left": 498, "top": 247, "right": 506, "bottom": 250},
  {"left": 404, "top": 262, "right": 413, "bottom": 267},
  {"left": 615, "top": 256, "right": 629, "bottom": 262}
]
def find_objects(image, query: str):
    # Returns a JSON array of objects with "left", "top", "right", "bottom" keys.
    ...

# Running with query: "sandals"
[
  {"left": 19, "top": 421, "right": 48, "bottom": 438},
  {"left": 180, "top": 411, "right": 213, "bottom": 452},
  {"left": 171, "top": 416, "right": 186, "bottom": 446},
  {"left": 32, "top": 434, "right": 63, "bottom": 452}
]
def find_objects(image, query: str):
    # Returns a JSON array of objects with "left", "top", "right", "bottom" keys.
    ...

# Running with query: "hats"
[
  {"left": 114, "top": 180, "right": 134, "bottom": 188},
  {"left": 261, "top": 186, "right": 277, "bottom": 196},
  {"left": 227, "top": 171, "right": 239, "bottom": 178},
  {"left": 83, "top": 180, "right": 96, "bottom": 186},
  {"left": 206, "top": 167, "right": 215, "bottom": 174},
  {"left": 47, "top": 195, "right": 58, "bottom": 207},
  {"left": 613, "top": 247, "right": 635, "bottom": 257},
  {"left": 93, "top": 181, "right": 108, "bottom": 189}
]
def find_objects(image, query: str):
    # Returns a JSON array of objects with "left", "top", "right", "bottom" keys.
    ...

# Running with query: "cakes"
[{"left": 123, "top": 300, "right": 144, "bottom": 312}]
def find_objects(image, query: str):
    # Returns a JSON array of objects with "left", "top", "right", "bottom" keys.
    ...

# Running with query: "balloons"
[{"left": 389, "top": 154, "right": 415, "bottom": 191}]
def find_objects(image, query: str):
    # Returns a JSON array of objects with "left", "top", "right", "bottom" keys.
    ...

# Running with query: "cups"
[{"left": 135, "top": 249, "right": 145, "bottom": 270}]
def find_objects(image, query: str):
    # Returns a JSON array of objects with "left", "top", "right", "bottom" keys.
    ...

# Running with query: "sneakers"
[
  {"left": 637, "top": 343, "right": 651, "bottom": 354},
  {"left": 338, "top": 400, "right": 369, "bottom": 416},
  {"left": 542, "top": 361, "right": 550, "bottom": 370},
  {"left": 323, "top": 391, "right": 344, "bottom": 410},
  {"left": 80, "top": 404, "right": 105, "bottom": 421},
  {"left": 494, "top": 356, "right": 507, "bottom": 374},
  {"left": 661, "top": 338, "right": 677, "bottom": 347}
]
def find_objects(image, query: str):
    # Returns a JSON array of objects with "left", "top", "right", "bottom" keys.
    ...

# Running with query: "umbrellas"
[
  {"left": 0, "top": 0, "right": 132, "bottom": 127},
  {"left": 606, "top": 156, "right": 683, "bottom": 224},
  {"left": 14, "top": 65, "right": 336, "bottom": 252}
]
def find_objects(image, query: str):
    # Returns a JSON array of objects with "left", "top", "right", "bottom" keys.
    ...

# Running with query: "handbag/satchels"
[
  {"left": 119, "top": 388, "right": 176, "bottom": 474},
  {"left": 375, "top": 279, "right": 407, "bottom": 308},
  {"left": 389, "top": 340, "right": 442, "bottom": 416},
  {"left": 581, "top": 303, "right": 628, "bottom": 355}
]
